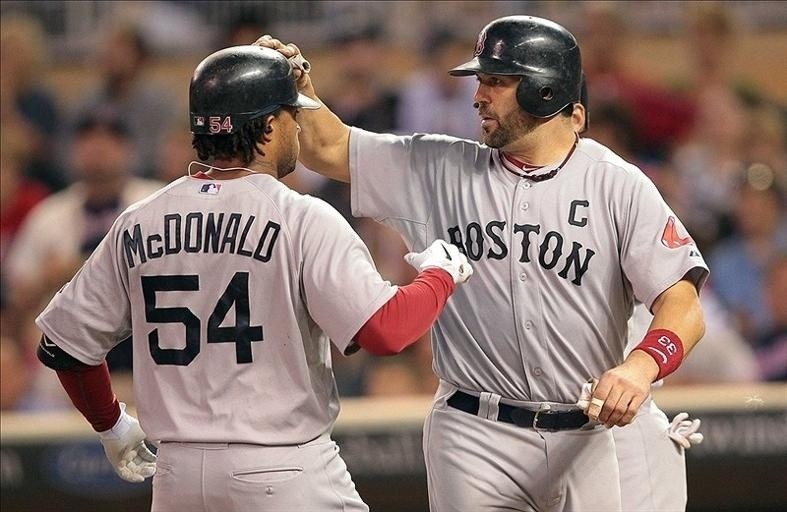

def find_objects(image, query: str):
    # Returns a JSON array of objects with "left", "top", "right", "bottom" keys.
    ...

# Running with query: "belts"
[{"left": 447, "top": 390, "right": 589, "bottom": 431}]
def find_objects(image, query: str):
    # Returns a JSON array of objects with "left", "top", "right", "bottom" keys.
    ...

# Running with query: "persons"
[
  {"left": 34, "top": 45, "right": 474, "bottom": 512},
  {"left": 1, "top": 1, "right": 786, "bottom": 410},
  {"left": 252, "top": 12, "right": 711, "bottom": 512},
  {"left": 580, "top": 69, "right": 688, "bottom": 510}
]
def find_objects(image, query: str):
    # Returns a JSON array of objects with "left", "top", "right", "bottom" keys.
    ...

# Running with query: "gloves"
[
  {"left": 669, "top": 413, "right": 704, "bottom": 450},
  {"left": 404, "top": 238, "right": 473, "bottom": 285},
  {"left": 94, "top": 402, "right": 161, "bottom": 483}
]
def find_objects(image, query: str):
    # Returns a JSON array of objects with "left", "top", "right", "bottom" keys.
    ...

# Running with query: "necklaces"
[{"left": 499, "top": 130, "right": 581, "bottom": 184}]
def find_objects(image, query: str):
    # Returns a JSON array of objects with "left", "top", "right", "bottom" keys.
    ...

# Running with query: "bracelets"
[{"left": 633, "top": 328, "right": 687, "bottom": 385}]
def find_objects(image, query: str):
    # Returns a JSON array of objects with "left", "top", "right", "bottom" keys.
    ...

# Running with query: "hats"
[{"left": 447, "top": 15, "right": 581, "bottom": 120}]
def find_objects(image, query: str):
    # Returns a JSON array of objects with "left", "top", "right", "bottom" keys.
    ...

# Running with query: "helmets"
[{"left": 188, "top": 45, "right": 321, "bottom": 134}]
